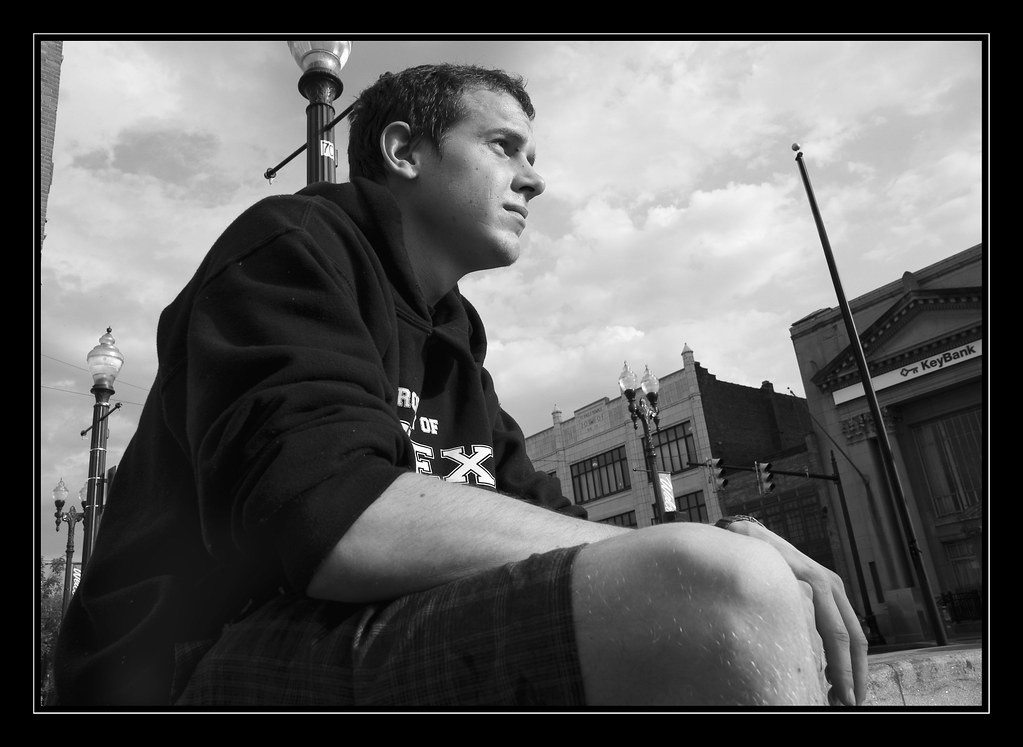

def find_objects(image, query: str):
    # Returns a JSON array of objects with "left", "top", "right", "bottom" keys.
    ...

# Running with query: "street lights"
[
  {"left": 52, "top": 477, "right": 90, "bottom": 630},
  {"left": 80, "top": 326, "right": 126, "bottom": 585},
  {"left": 285, "top": 40, "right": 357, "bottom": 189},
  {"left": 617, "top": 361, "right": 670, "bottom": 525}
]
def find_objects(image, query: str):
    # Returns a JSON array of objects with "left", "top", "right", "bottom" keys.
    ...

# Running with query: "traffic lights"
[
  {"left": 708, "top": 458, "right": 728, "bottom": 492},
  {"left": 755, "top": 461, "right": 776, "bottom": 495}
]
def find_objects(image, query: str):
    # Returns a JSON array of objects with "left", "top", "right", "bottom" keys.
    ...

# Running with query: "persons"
[{"left": 50, "top": 63, "right": 869, "bottom": 708}]
